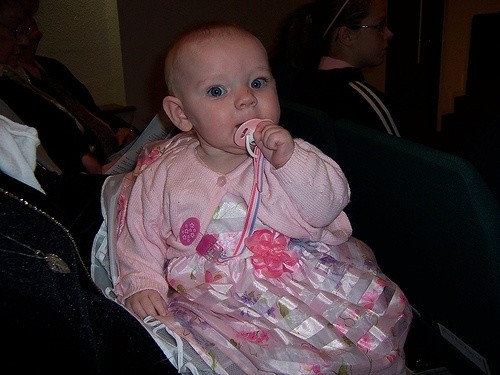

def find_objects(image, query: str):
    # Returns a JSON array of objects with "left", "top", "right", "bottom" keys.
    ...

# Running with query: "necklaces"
[{"left": 0, "top": 231, "right": 71, "bottom": 276}]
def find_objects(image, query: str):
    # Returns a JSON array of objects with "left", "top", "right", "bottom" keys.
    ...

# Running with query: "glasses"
[
  {"left": 352, "top": 22, "right": 386, "bottom": 32},
  {"left": 6, "top": 26, "right": 32, "bottom": 37}
]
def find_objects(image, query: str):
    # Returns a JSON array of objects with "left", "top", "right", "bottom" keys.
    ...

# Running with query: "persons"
[
  {"left": 0, "top": 164, "right": 182, "bottom": 374},
  {"left": 21, "top": 14, "right": 139, "bottom": 153},
  {"left": 115, "top": 25, "right": 411, "bottom": 375},
  {"left": 0, "top": 1, "right": 114, "bottom": 193},
  {"left": 273, "top": 1, "right": 411, "bottom": 141}
]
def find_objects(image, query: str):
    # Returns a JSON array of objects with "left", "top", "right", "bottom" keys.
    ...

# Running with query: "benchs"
[{"left": 277, "top": 99, "right": 500, "bottom": 354}]
